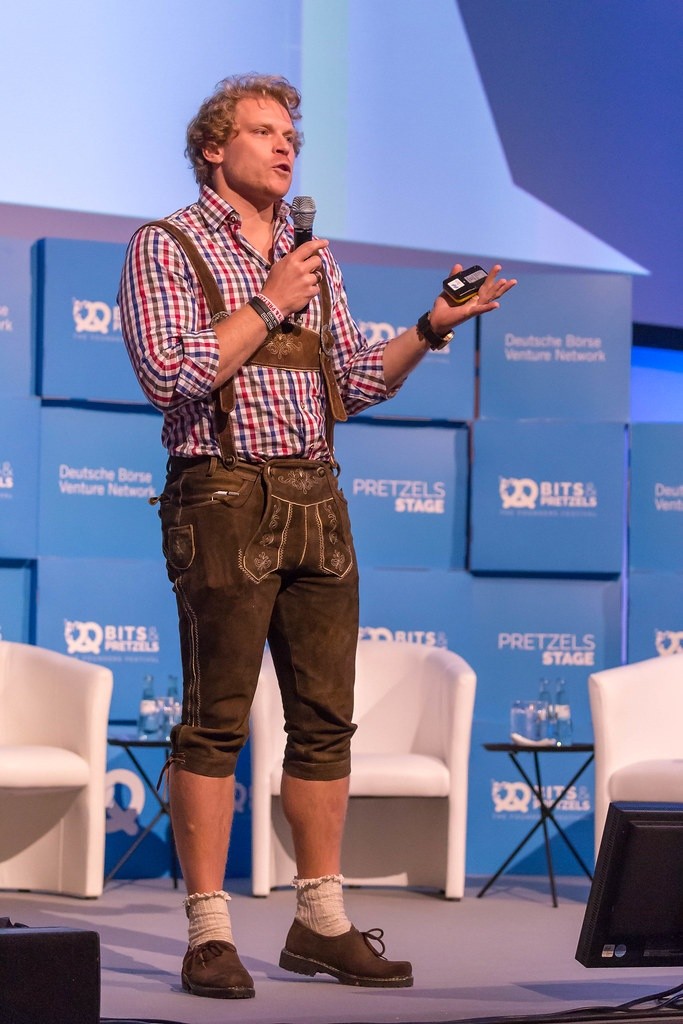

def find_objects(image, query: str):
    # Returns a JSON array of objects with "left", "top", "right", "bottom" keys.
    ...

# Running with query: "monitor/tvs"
[{"left": 573, "top": 799, "right": 683, "bottom": 968}]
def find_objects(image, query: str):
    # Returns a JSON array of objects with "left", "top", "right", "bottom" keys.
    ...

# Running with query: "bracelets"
[{"left": 245, "top": 293, "right": 284, "bottom": 332}]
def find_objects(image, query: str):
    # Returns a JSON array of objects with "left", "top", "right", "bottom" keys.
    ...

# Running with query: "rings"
[{"left": 312, "top": 270, "right": 321, "bottom": 283}]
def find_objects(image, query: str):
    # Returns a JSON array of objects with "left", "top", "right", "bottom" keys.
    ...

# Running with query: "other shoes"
[
  {"left": 182, "top": 939, "right": 254, "bottom": 997},
  {"left": 280, "top": 918, "right": 412, "bottom": 989}
]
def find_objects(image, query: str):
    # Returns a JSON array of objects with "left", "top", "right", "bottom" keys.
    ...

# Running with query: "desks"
[
  {"left": 107, "top": 733, "right": 182, "bottom": 892},
  {"left": 474, "top": 740, "right": 596, "bottom": 909}
]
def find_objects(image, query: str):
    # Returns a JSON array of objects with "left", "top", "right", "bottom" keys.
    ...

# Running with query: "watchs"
[{"left": 417, "top": 310, "right": 455, "bottom": 352}]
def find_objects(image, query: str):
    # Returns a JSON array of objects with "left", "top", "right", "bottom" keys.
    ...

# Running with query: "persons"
[{"left": 117, "top": 70, "right": 519, "bottom": 1002}]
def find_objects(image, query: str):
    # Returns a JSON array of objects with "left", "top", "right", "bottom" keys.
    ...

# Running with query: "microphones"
[{"left": 289, "top": 195, "right": 317, "bottom": 313}]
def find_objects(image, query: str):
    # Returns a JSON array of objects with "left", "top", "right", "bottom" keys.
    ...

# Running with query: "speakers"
[{"left": 0, "top": 925, "right": 101, "bottom": 1024}]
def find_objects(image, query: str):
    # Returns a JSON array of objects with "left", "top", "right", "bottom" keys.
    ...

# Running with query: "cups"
[{"left": 510, "top": 701, "right": 535, "bottom": 743}]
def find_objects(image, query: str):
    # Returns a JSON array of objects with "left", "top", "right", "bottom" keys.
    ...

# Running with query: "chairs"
[
  {"left": 589, "top": 653, "right": 683, "bottom": 878},
  {"left": 246, "top": 639, "right": 477, "bottom": 900},
  {"left": 0, "top": 638, "right": 114, "bottom": 900}
]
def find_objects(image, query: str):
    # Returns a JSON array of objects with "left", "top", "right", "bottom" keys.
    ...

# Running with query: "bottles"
[
  {"left": 554, "top": 678, "right": 573, "bottom": 747},
  {"left": 164, "top": 676, "right": 182, "bottom": 737},
  {"left": 136, "top": 676, "right": 163, "bottom": 741},
  {"left": 535, "top": 678, "right": 552, "bottom": 744}
]
